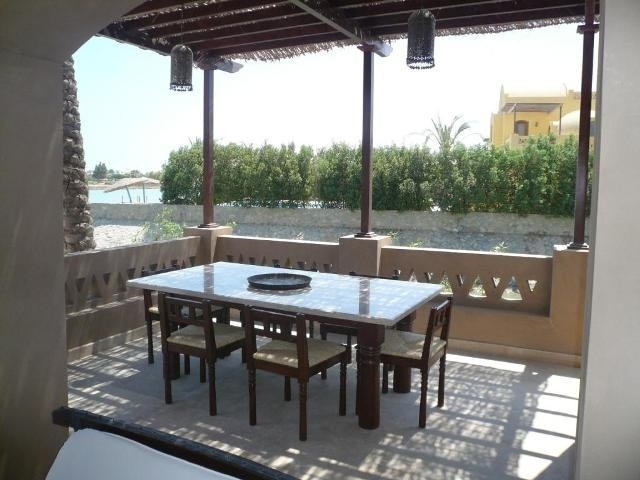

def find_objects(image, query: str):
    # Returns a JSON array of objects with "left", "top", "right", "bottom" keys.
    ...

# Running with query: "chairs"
[
  {"left": 319, "top": 270, "right": 400, "bottom": 380},
  {"left": 144, "top": 289, "right": 227, "bottom": 364},
  {"left": 356, "top": 296, "right": 453, "bottom": 428},
  {"left": 158, "top": 293, "right": 245, "bottom": 416},
  {"left": 244, "top": 305, "right": 347, "bottom": 441},
  {"left": 240, "top": 308, "right": 314, "bottom": 352}
]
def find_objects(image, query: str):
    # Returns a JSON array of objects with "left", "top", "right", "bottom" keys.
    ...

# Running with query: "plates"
[{"left": 247, "top": 273, "right": 312, "bottom": 290}]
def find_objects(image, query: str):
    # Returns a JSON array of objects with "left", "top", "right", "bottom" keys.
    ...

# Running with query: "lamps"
[
  {"left": 407, "top": 9, "right": 435, "bottom": 69},
  {"left": 171, "top": 45, "right": 193, "bottom": 91}
]
